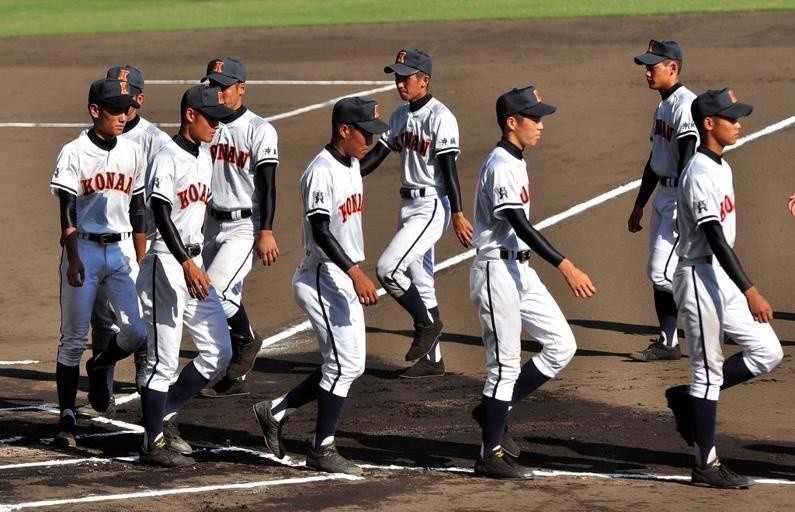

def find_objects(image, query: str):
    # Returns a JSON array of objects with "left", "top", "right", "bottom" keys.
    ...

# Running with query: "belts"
[
  {"left": 658, "top": 175, "right": 679, "bottom": 188},
  {"left": 77, "top": 231, "right": 131, "bottom": 243},
  {"left": 150, "top": 241, "right": 205, "bottom": 257},
  {"left": 477, "top": 248, "right": 532, "bottom": 263},
  {"left": 208, "top": 207, "right": 258, "bottom": 221},
  {"left": 679, "top": 255, "right": 721, "bottom": 265},
  {"left": 399, "top": 186, "right": 446, "bottom": 197}
]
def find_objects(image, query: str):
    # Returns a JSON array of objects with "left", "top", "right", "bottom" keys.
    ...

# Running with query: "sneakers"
[
  {"left": 161, "top": 415, "right": 192, "bottom": 455},
  {"left": 630, "top": 335, "right": 682, "bottom": 362},
  {"left": 77, "top": 396, "right": 116, "bottom": 419},
  {"left": 472, "top": 403, "right": 519, "bottom": 458},
  {"left": 399, "top": 358, "right": 446, "bottom": 377},
  {"left": 85, "top": 357, "right": 110, "bottom": 412},
  {"left": 305, "top": 439, "right": 363, "bottom": 476},
  {"left": 689, "top": 456, "right": 757, "bottom": 488},
  {"left": 53, "top": 416, "right": 76, "bottom": 450},
  {"left": 139, "top": 437, "right": 195, "bottom": 468},
  {"left": 253, "top": 401, "right": 289, "bottom": 459},
  {"left": 224, "top": 331, "right": 262, "bottom": 380},
  {"left": 665, "top": 384, "right": 694, "bottom": 449},
  {"left": 474, "top": 446, "right": 535, "bottom": 480},
  {"left": 133, "top": 360, "right": 147, "bottom": 395},
  {"left": 198, "top": 376, "right": 251, "bottom": 399},
  {"left": 405, "top": 315, "right": 445, "bottom": 362}
]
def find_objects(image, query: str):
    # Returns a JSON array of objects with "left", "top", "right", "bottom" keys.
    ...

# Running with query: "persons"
[
  {"left": 359, "top": 49, "right": 474, "bottom": 381},
  {"left": 627, "top": 38, "right": 701, "bottom": 362},
  {"left": 471, "top": 85, "right": 598, "bottom": 481},
  {"left": 194, "top": 56, "right": 280, "bottom": 398},
  {"left": 665, "top": 86, "right": 784, "bottom": 489},
  {"left": 252, "top": 96, "right": 391, "bottom": 475},
  {"left": 135, "top": 85, "right": 233, "bottom": 468},
  {"left": 90, "top": 64, "right": 172, "bottom": 393},
  {"left": 49, "top": 77, "right": 147, "bottom": 450},
  {"left": 786, "top": 193, "right": 795, "bottom": 218}
]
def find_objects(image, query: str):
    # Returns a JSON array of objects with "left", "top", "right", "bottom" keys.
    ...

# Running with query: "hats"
[
  {"left": 333, "top": 98, "right": 389, "bottom": 134},
  {"left": 495, "top": 87, "right": 557, "bottom": 119},
  {"left": 107, "top": 65, "right": 145, "bottom": 95},
  {"left": 383, "top": 47, "right": 434, "bottom": 77},
  {"left": 633, "top": 39, "right": 682, "bottom": 66},
  {"left": 89, "top": 79, "right": 140, "bottom": 110},
  {"left": 690, "top": 89, "right": 754, "bottom": 120},
  {"left": 182, "top": 85, "right": 237, "bottom": 120},
  {"left": 200, "top": 56, "right": 247, "bottom": 86}
]
